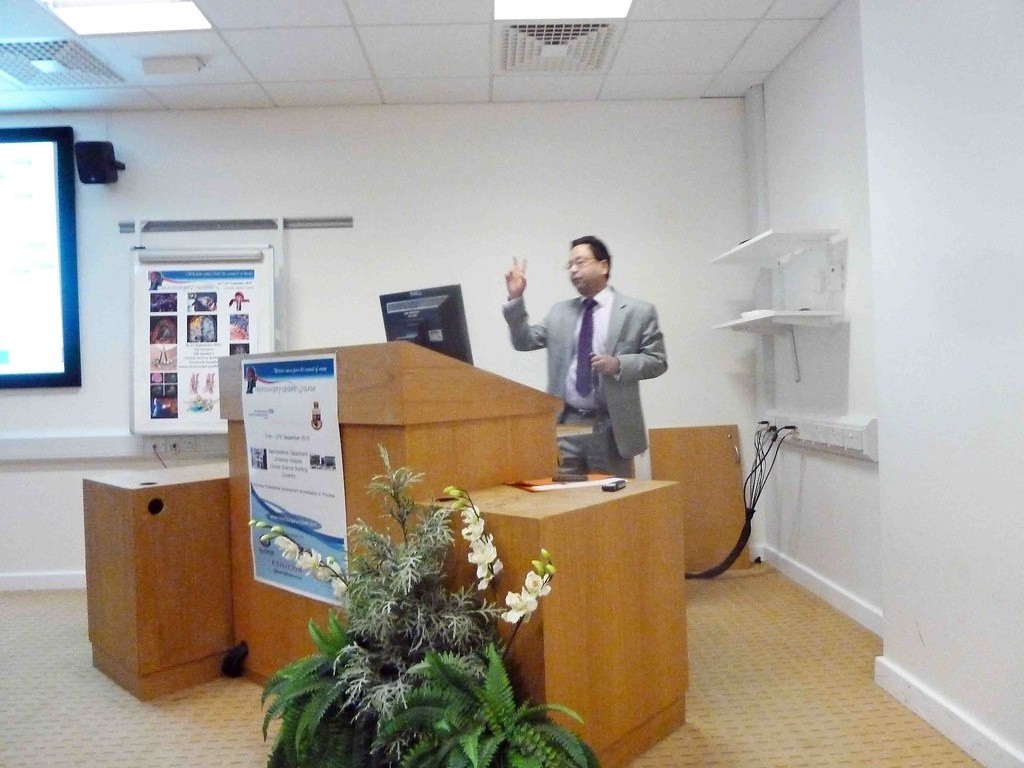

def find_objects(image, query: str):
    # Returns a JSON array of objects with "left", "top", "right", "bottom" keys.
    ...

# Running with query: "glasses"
[{"left": 567, "top": 257, "right": 597, "bottom": 269}]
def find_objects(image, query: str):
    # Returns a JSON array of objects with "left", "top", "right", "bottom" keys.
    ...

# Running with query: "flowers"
[{"left": 263, "top": 439, "right": 599, "bottom": 768}]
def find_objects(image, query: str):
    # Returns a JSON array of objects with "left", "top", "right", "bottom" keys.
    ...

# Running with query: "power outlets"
[
  {"left": 145, "top": 437, "right": 197, "bottom": 451},
  {"left": 757, "top": 415, "right": 876, "bottom": 465}
]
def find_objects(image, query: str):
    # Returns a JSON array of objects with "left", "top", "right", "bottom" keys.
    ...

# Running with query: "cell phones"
[{"left": 599, "top": 478, "right": 626, "bottom": 492}]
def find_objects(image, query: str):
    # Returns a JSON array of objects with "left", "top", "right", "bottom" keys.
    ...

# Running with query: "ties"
[{"left": 575, "top": 298, "right": 598, "bottom": 399}]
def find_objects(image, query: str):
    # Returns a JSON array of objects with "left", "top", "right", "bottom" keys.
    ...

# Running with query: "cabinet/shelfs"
[{"left": 711, "top": 229, "right": 845, "bottom": 337}]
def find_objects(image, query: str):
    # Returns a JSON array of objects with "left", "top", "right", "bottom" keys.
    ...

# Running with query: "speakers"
[{"left": 73, "top": 140, "right": 119, "bottom": 185}]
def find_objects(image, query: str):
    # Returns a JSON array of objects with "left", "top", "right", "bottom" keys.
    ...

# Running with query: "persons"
[{"left": 502, "top": 235, "right": 669, "bottom": 478}]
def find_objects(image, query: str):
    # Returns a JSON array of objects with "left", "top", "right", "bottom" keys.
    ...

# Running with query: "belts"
[{"left": 565, "top": 403, "right": 608, "bottom": 418}]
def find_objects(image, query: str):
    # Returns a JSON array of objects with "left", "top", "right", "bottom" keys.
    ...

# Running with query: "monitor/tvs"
[{"left": 377, "top": 282, "right": 475, "bottom": 367}]
